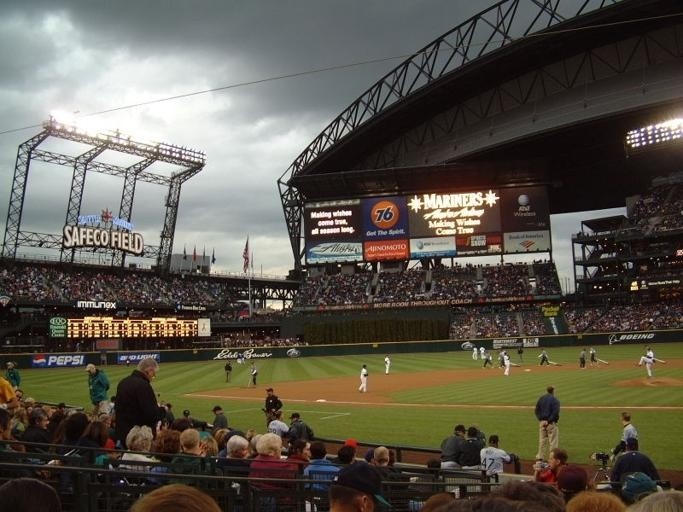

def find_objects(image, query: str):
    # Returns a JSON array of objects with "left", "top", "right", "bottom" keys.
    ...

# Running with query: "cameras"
[{"left": 160, "top": 400, "right": 173, "bottom": 412}]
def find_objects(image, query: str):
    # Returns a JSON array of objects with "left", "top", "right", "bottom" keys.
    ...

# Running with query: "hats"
[
  {"left": 289, "top": 413, "right": 300, "bottom": 418},
  {"left": 84, "top": 364, "right": 95, "bottom": 371},
  {"left": 266, "top": 388, "right": 273, "bottom": 392},
  {"left": 332, "top": 462, "right": 394, "bottom": 508},
  {"left": 374, "top": 446, "right": 389, "bottom": 460},
  {"left": 184, "top": 410, "right": 190, "bottom": 416},
  {"left": 210, "top": 406, "right": 221, "bottom": 411},
  {"left": 345, "top": 439, "right": 359, "bottom": 448},
  {"left": 620, "top": 471, "right": 657, "bottom": 504},
  {"left": 6, "top": 362, "right": 14, "bottom": 367},
  {"left": 455, "top": 424, "right": 498, "bottom": 442}
]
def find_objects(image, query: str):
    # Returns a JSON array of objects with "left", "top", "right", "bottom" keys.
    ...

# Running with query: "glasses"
[{"left": 16, "top": 395, "right": 22, "bottom": 398}]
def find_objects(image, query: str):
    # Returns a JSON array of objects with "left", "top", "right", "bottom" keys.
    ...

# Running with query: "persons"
[
  {"left": 308, "top": 183, "right": 683, "bottom": 340},
  {"left": 224, "top": 357, "right": 232, "bottom": 382},
  {"left": 247, "top": 360, "right": 258, "bottom": 388},
  {"left": 1, "top": 262, "right": 305, "bottom": 350},
  {"left": 634, "top": 345, "right": 655, "bottom": 380},
  {"left": 263, "top": 386, "right": 283, "bottom": 427},
  {"left": 384, "top": 354, "right": 391, "bottom": 375},
  {"left": 85, "top": 360, "right": 111, "bottom": 408},
  {"left": 358, "top": 364, "right": 369, "bottom": 393},
  {"left": 610, "top": 412, "right": 640, "bottom": 455},
  {"left": 0, "top": 338, "right": 682, "bottom": 512},
  {"left": 534, "top": 382, "right": 561, "bottom": 461},
  {"left": 115, "top": 359, "right": 170, "bottom": 446}
]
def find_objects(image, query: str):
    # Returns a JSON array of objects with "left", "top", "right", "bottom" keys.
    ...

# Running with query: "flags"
[
  {"left": 180, "top": 242, "right": 219, "bottom": 264},
  {"left": 239, "top": 233, "right": 257, "bottom": 276}
]
